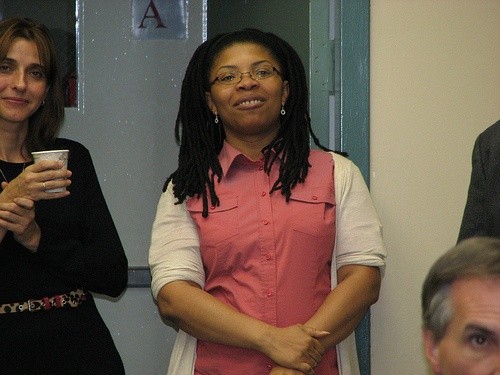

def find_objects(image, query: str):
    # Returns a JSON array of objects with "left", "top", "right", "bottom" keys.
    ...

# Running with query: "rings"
[{"left": 42, "top": 182, "right": 46, "bottom": 190}]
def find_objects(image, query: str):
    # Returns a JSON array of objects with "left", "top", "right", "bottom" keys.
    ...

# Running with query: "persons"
[
  {"left": 421, "top": 119, "right": 500, "bottom": 375},
  {"left": 148, "top": 27, "right": 385, "bottom": 375},
  {"left": 0, "top": 18, "right": 128, "bottom": 375}
]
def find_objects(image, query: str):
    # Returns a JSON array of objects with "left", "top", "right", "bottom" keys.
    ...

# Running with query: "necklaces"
[{"left": 0, "top": 155, "right": 27, "bottom": 184}]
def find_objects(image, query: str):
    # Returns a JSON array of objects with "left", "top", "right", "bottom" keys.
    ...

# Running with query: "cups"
[{"left": 30, "top": 150, "right": 68, "bottom": 194}]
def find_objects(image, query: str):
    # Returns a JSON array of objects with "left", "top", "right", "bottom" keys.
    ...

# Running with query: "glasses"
[{"left": 209, "top": 67, "right": 283, "bottom": 87}]
